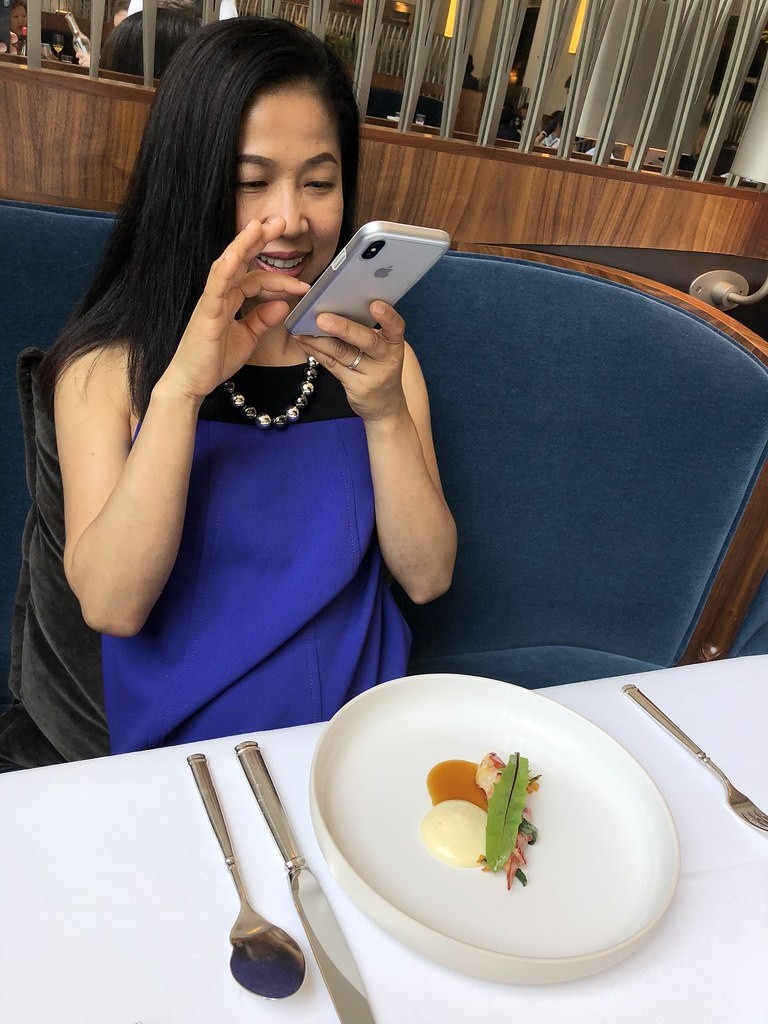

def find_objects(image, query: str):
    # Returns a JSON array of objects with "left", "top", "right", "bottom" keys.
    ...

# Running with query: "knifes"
[{"left": 235, "top": 741, "right": 373, "bottom": 1023}]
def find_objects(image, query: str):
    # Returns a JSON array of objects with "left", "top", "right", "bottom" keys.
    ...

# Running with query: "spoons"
[{"left": 179, "top": 754, "right": 305, "bottom": 999}]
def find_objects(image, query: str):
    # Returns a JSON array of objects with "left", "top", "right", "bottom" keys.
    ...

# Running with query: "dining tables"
[{"left": 0, "top": 653, "right": 767, "bottom": 1024}]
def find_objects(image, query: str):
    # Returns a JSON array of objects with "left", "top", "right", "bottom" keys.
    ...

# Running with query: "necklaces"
[{"left": 223, "top": 356, "right": 319, "bottom": 429}]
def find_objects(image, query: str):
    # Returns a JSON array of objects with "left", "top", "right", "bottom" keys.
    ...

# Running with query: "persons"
[
  {"left": 462, "top": 53, "right": 596, "bottom": 161},
  {"left": 54, "top": 17, "right": 459, "bottom": 754},
  {"left": 0, "top": 0, "right": 203, "bottom": 79}
]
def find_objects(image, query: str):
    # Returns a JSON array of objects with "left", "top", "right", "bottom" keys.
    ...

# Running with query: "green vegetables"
[{"left": 484, "top": 751, "right": 539, "bottom": 887}]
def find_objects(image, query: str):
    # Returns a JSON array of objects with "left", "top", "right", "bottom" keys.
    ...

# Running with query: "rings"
[{"left": 348, "top": 351, "right": 363, "bottom": 370}]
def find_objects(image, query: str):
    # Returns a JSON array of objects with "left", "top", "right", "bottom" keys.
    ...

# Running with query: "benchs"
[
  {"left": 1, "top": 189, "right": 768, "bottom": 716},
  {"left": 364, "top": 85, "right": 446, "bottom": 129}
]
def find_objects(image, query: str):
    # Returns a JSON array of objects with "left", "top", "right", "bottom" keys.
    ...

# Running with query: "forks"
[{"left": 621, "top": 684, "right": 768, "bottom": 832}]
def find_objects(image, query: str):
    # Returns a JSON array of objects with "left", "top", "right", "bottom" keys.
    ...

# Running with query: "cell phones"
[{"left": 283, "top": 221, "right": 452, "bottom": 338}]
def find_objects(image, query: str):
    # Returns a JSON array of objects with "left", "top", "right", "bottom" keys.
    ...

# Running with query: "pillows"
[{"left": 0, "top": 346, "right": 114, "bottom": 773}]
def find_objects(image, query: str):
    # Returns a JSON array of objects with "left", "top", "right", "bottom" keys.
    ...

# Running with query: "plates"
[{"left": 308, "top": 674, "right": 680, "bottom": 986}]
